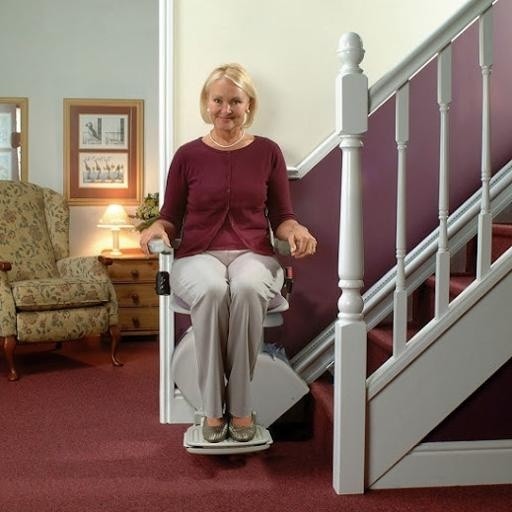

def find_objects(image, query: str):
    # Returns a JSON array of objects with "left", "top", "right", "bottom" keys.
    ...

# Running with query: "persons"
[{"left": 140, "top": 64, "right": 320, "bottom": 442}]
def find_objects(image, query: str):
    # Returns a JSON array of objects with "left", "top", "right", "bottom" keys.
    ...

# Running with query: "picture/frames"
[{"left": 62, "top": 97, "right": 147, "bottom": 208}]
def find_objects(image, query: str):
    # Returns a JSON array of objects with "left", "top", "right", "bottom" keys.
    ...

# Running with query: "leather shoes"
[
  {"left": 202, "top": 411, "right": 228, "bottom": 443},
  {"left": 229, "top": 415, "right": 257, "bottom": 442}
]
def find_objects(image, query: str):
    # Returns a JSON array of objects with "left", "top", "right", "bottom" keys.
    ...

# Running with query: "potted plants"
[{"left": 131, "top": 190, "right": 159, "bottom": 250}]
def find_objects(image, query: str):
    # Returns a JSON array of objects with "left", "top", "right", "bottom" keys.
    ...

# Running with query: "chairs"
[{"left": 149, "top": 222, "right": 287, "bottom": 457}]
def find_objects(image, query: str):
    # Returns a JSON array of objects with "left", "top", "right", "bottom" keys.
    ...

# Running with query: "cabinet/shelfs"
[{"left": 99, "top": 252, "right": 163, "bottom": 336}]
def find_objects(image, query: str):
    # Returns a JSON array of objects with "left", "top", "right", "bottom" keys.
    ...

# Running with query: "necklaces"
[{"left": 206, "top": 129, "right": 248, "bottom": 147}]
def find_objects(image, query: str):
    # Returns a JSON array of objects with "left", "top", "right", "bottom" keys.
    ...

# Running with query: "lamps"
[{"left": 95, "top": 202, "right": 134, "bottom": 252}]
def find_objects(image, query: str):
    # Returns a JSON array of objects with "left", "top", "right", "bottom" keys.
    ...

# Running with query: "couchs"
[{"left": 0, "top": 179, "right": 126, "bottom": 379}]
